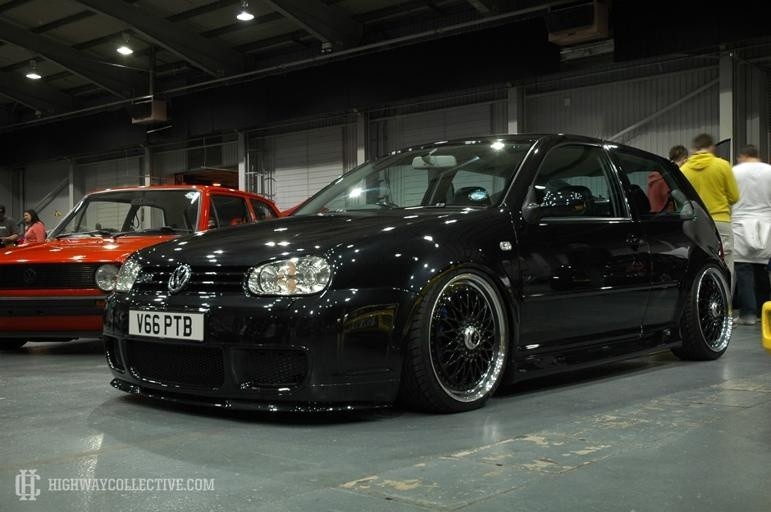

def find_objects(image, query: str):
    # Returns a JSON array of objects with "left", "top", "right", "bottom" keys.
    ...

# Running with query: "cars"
[
  {"left": 100, "top": 131, "right": 734, "bottom": 416},
  {"left": 0, "top": 185, "right": 330, "bottom": 353}
]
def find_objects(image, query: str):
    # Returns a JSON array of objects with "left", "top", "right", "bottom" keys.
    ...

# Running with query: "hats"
[{"left": 0, "top": 206, "right": 6, "bottom": 213}]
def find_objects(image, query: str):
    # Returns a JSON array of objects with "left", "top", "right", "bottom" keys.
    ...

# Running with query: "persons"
[
  {"left": 0, "top": 204, "right": 24, "bottom": 249},
  {"left": 647, "top": 143, "right": 688, "bottom": 212},
  {"left": 679, "top": 133, "right": 741, "bottom": 327},
  {"left": 23, "top": 208, "right": 45, "bottom": 243},
  {"left": 731, "top": 144, "right": 771, "bottom": 324}
]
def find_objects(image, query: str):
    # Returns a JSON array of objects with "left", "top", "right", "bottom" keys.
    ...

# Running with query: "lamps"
[
  {"left": 236, "top": 0, "right": 255, "bottom": 21},
  {"left": 116, "top": 38, "right": 134, "bottom": 56},
  {"left": 25, "top": 60, "right": 43, "bottom": 79}
]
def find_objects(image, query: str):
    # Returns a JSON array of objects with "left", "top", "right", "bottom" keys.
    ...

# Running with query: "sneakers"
[{"left": 733, "top": 314, "right": 757, "bottom": 324}]
